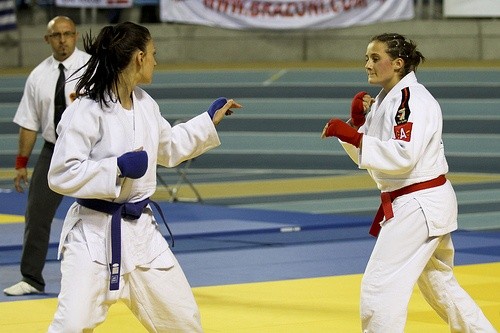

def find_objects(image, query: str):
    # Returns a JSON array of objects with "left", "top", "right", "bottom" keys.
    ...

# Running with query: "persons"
[
  {"left": 3, "top": 15, "right": 94, "bottom": 296},
  {"left": 47, "top": 21, "right": 242, "bottom": 333},
  {"left": 322, "top": 33, "right": 497, "bottom": 333}
]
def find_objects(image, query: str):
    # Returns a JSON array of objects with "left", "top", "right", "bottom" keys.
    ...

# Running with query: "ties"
[{"left": 54, "top": 63, "right": 66, "bottom": 140}]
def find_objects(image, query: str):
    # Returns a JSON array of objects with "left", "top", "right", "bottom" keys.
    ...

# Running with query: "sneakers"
[{"left": 3, "top": 281, "right": 43, "bottom": 296}]
